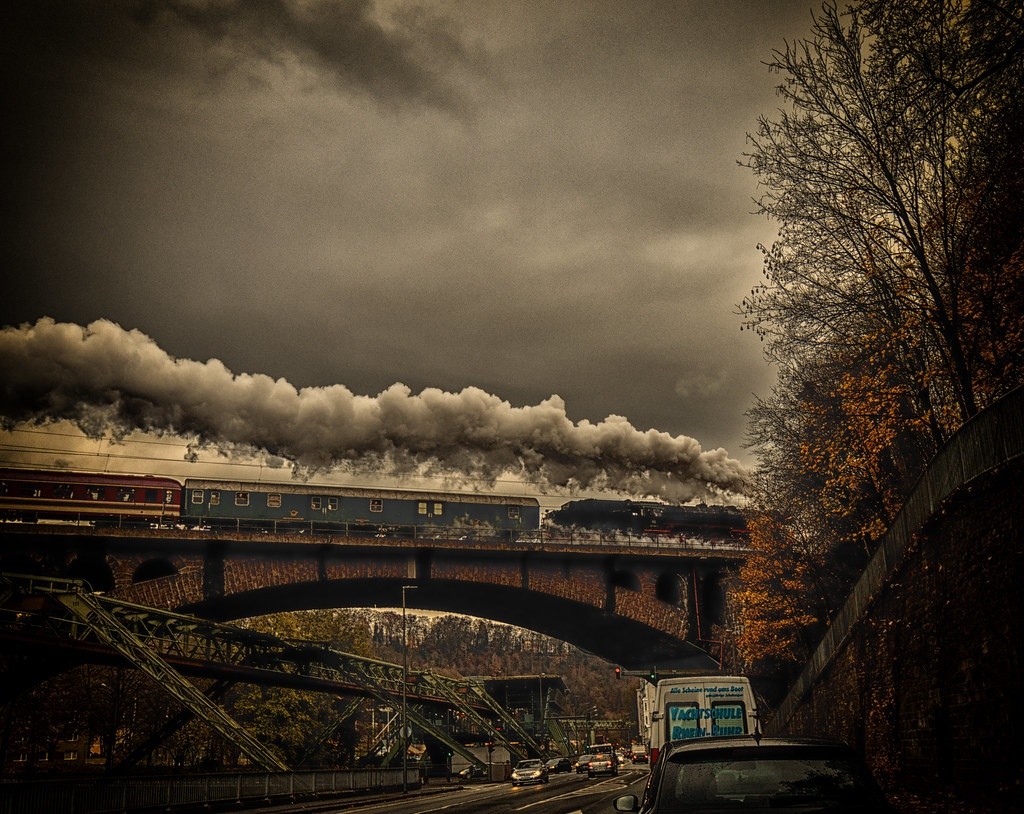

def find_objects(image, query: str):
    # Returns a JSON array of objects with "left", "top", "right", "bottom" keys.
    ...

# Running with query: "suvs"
[
  {"left": 511, "top": 759, "right": 549, "bottom": 787},
  {"left": 611, "top": 731, "right": 901, "bottom": 814}
]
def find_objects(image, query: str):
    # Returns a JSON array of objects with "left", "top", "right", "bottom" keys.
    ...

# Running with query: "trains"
[{"left": 1, "top": 466, "right": 795, "bottom": 550}]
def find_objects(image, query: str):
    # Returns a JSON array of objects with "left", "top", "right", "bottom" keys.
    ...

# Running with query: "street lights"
[
  {"left": 401, "top": 585, "right": 419, "bottom": 793},
  {"left": 539, "top": 673, "right": 546, "bottom": 744}
]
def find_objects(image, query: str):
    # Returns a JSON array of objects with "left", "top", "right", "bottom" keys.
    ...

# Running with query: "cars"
[
  {"left": 545, "top": 757, "right": 572, "bottom": 774},
  {"left": 575, "top": 755, "right": 593, "bottom": 774},
  {"left": 458, "top": 762, "right": 495, "bottom": 781},
  {"left": 632, "top": 751, "right": 648, "bottom": 765},
  {"left": 588, "top": 754, "right": 618, "bottom": 778},
  {"left": 616, "top": 752, "right": 624, "bottom": 764}
]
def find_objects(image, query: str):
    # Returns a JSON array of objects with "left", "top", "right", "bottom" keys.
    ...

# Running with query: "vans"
[{"left": 649, "top": 677, "right": 764, "bottom": 773}]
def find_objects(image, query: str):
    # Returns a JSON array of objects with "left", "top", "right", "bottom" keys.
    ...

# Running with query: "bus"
[{"left": 586, "top": 743, "right": 612, "bottom": 755}]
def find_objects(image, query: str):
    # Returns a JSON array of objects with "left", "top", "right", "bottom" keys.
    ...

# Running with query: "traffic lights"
[
  {"left": 614, "top": 666, "right": 621, "bottom": 679},
  {"left": 650, "top": 666, "right": 657, "bottom": 680}
]
